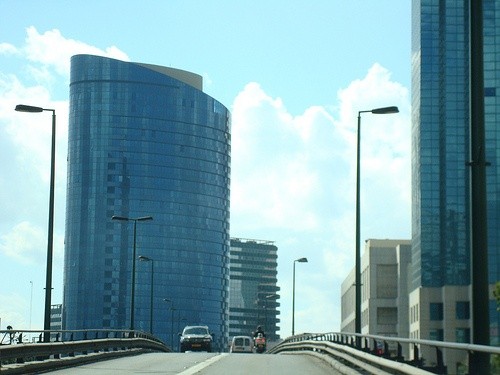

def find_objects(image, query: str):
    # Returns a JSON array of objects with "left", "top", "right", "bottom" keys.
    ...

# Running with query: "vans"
[{"left": 230, "top": 336, "right": 255, "bottom": 353}]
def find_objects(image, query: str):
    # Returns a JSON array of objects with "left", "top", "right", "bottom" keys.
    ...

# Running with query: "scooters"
[{"left": 252, "top": 337, "right": 266, "bottom": 354}]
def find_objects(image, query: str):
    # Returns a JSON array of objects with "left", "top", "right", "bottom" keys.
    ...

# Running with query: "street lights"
[
  {"left": 138, "top": 255, "right": 154, "bottom": 336},
  {"left": 14, "top": 103, "right": 57, "bottom": 343},
  {"left": 354, "top": 105, "right": 401, "bottom": 350},
  {"left": 290, "top": 257, "right": 309, "bottom": 336},
  {"left": 112, "top": 214, "right": 154, "bottom": 338}
]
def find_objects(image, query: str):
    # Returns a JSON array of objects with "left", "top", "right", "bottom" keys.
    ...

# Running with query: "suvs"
[{"left": 178, "top": 325, "right": 215, "bottom": 353}]
